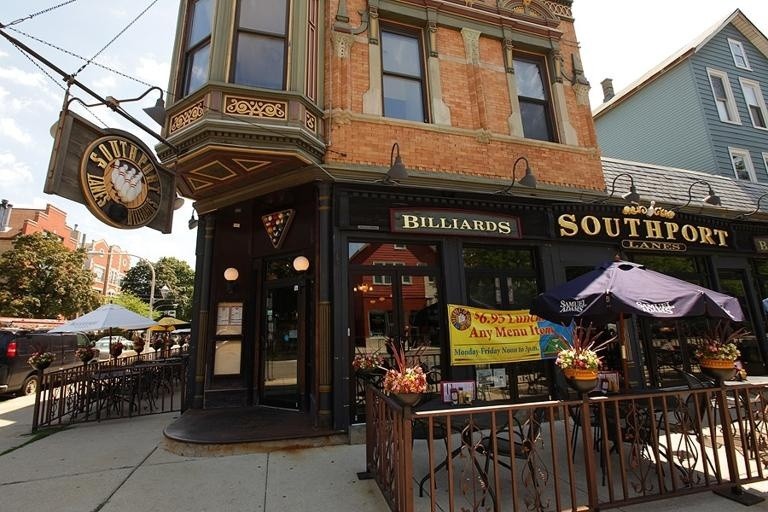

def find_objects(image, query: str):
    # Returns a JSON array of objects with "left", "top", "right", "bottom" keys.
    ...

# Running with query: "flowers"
[
  {"left": 687, "top": 319, "right": 752, "bottom": 360},
  {"left": 351, "top": 353, "right": 384, "bottom": 372},
  {"left": 28, "top": 336, "right": 144, "bottom": 370},
  {"left": 383, "top": 336, "right": 431, "bottom": 392},
  {"left": 547, "top": 318, "right": 617, "bottom": 368}
]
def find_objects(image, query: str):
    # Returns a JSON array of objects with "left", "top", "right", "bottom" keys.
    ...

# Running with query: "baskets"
[
  {"left": 700, "top": 358, "right": 736, "bottom": 370},
  {"left": 561, "top": 367, "right": 598, "bottom": 380}
]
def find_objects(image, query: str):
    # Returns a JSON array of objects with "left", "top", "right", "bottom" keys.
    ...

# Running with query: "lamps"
[
  {"left": 222, "top": 265, "right": 241, "bottom": 293},
  {"left": 491, "top": 155, "right": 538, "bottom": 194},
  {"left": 292, "top": 255, "right": 313, "bottom": 286},
  {"left": 188, "top": 207, "right": 199, "bottom": 230},
  {"left": 671, "top": 178, "right": 724, "bottom": 210},
  {"left": 64, "top": 86, "right": 168, "bottom": 128},
  {"left": 585, "top": 173, "right": 643, "bottom": 206},
  {"left": 384, "top": 142, "right": 408, "bottom": 184}
]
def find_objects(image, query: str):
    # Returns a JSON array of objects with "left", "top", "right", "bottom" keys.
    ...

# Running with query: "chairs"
[
  {"left": 564, "top": 368, "right": 758, "bottom": 486},
  {"left": 478, "top": 408, "right": 548, "bottom": 510},
  {"left": 73, "top": 352, "right": 191, "bottom": 416}
]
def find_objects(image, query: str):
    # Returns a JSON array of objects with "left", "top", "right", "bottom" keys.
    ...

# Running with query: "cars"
[{"left": 95, "top": 335, "right": 134, "bottom": 354}]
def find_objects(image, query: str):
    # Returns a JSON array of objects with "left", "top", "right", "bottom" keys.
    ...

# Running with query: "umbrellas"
[
  {"left": 47, "top": 300, "right": 158, "bottom": 354},
  {"left": 530, "top": 254, "right": 746, "bottom": 389},
  {"left": 158, "top": 316, "right": 190, "bottom": 331}
]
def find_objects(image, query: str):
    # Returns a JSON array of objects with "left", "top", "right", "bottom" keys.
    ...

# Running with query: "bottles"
[
  {"left": 450, "top": 388, "right": 472, "bottom": 404},
  {"left": 477, "top": 383, "right": 491, "bottom": 401}
]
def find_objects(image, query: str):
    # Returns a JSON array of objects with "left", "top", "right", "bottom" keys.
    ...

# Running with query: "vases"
[
  {"left": 360, "top": 367, "right": 374, "bottom": 374},
  {"left": 393, "top": 390, "right": 422, "bottom": 407},
  {"left": 699, "top": 359, "right": 734, "bottom": 379}
]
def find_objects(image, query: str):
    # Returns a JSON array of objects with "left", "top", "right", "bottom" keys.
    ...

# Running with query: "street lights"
[{"left": 86, "top": 251, "right": 178, "bottom": 354}]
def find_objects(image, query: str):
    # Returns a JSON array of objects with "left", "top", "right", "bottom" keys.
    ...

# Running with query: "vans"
[{"left": 0, "top": 328, "right": 100, "bottom": 396}]
